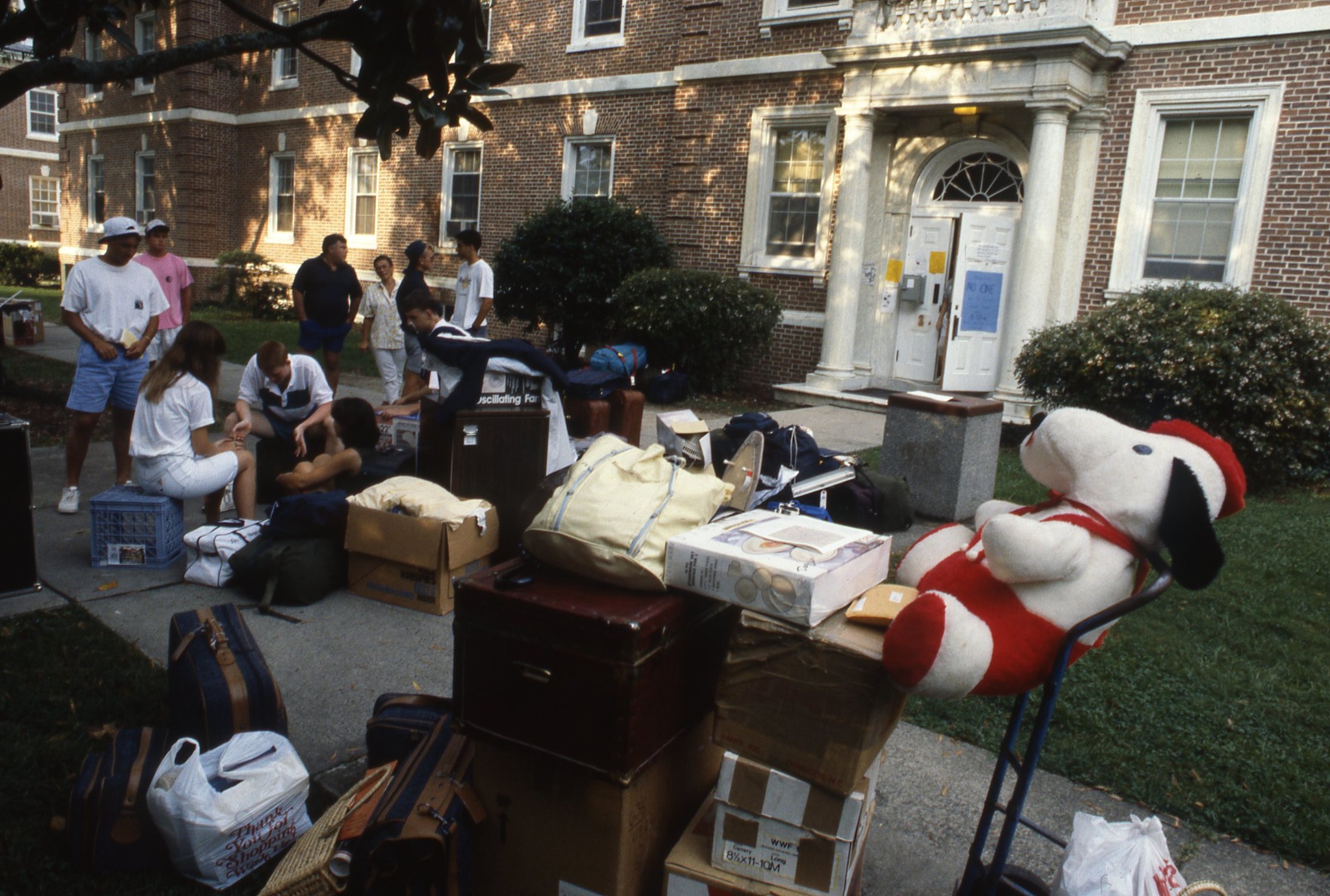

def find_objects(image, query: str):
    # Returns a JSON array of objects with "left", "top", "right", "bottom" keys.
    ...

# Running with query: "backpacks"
[{"left": 62, "top": 725, "right": 160, "bottom": 873}]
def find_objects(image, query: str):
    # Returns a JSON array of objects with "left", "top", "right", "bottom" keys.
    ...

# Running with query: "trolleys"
[{"left": 951, "top": 556, "right": 1182, "bottom": 896}]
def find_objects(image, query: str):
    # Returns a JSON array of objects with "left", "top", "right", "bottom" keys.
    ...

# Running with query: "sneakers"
[{"left": 57, "top": 486, "right": 80, "bottom": 514}]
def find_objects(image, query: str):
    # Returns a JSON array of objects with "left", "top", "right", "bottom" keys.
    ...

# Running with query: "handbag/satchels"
[
  {"left": 1054, "top": 809, "right": 1187, "bottom": 896},
  {"left": 230, "top": 533, "right": 344, "bottom": 607},
  {"left": 265, "top": 488, "right": 345, "bottom": 536},
  {"left": 531, "top": 431, "right": 732, "bottom": 590},
  {"left": 365, "top": 695, "right": 454, "bottom": 759},
  {"left": 590, "top": 343, "right": 647, "bottom": 382},
  {"left": 147, "top": 730, "right": 311, "bottom": 886},
  {"left": 182, "top": 517, "right": 261, "bottom": 587},
  {"left": 255, "top": 765, "right": 391, "bottom": 896},
  {"left": 710, "top": 412, "right": 915, "bottom": 531}
]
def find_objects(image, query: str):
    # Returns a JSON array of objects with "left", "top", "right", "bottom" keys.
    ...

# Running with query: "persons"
[
  {"left": 274, "top": 396, "right": 383, "bottom": 502},
  {"left": 380, "top": 287, "right": 475, "bottom": 423},
  {"left": 132, "top": 219, "right": 195, "bottom": 370},
  {"left": 128, "top": 320, "right": 258, "bottom": 521},
  {"left": 448, "top": 228, "right": 495, "bottom": 337},
  {"left": 358, "top": 256, "right": 408, "bottom": 407},
  {"left": 202, "top": 340, "right": 345, "bottom": 514},
  {"left": 56, "top": 215, "right": 172, "bottom": 514},
  {"left": 291, "top": 233, "right": 363, "bottom": 402},
  {"left": 395, "top": 241, "right": 437, "bottom": 403}
]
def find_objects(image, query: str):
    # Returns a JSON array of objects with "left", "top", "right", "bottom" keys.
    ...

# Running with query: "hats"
[
  {"left": 403, "top": 240, "right": 426, "bottom": 260},
  {"left": 145, "top": 218, "right": 170, "bottom": 235},
  {"left": 97, "top": 217, "right": 141, "bottom": 244}
]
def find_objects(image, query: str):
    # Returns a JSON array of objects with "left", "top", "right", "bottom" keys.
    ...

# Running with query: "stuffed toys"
[{"left": 882, "top": 405, "right": 1248, "bottom": 701}]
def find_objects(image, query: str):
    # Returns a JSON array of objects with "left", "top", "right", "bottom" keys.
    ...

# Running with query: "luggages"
[
  {"left": 168, "top": 603, "right": 288, "bottom": 742},
  {"left": 352, "top": 712, "right": 482, "bottom": 896},
  {"left": 350, "top": 443, "right": 416, "bottom": 494}
]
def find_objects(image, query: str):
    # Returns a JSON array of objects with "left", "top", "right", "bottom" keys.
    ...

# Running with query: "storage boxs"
[
  {"left": 0, "top": 382, "right": 917, "bottom": 896},
  {"left": 0, "top": 298, "right": 45, "bottom": 346}
]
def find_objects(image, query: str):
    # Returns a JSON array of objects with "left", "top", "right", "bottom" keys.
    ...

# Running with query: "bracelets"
[{"left": 141, "top": 335, "right": 152, "bottom": 344}]
